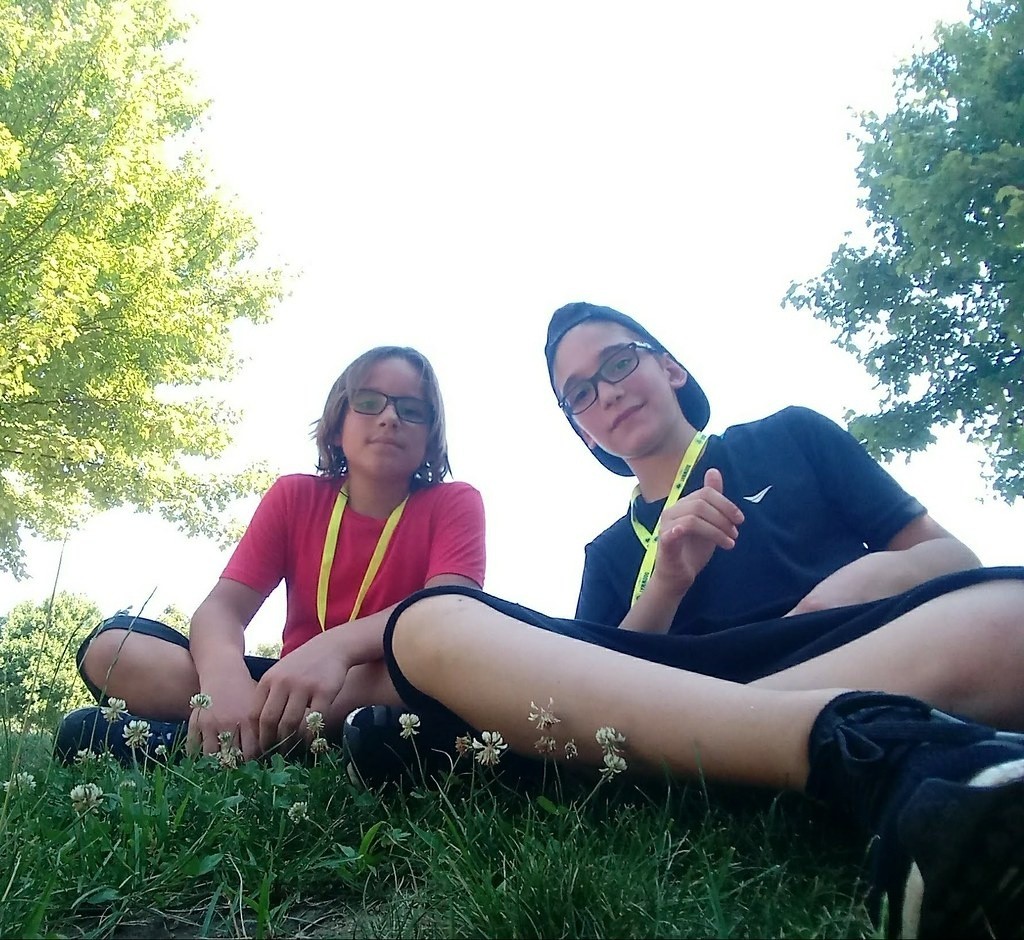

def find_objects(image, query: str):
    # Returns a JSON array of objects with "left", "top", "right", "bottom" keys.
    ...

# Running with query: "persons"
[
  {"left": 54, "top": 346, "right": 486, "bottom": 768},
  {"left": 340, "top": 302, "right": 1024, "bottom": 938}
]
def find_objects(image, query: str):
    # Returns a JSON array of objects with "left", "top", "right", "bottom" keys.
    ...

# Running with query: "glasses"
[
  {"left": 349, "top": 388, "right": 432, "bottom": 424},
  {"left": 558, "top": 341, "right": 655, "bottom": 414}
]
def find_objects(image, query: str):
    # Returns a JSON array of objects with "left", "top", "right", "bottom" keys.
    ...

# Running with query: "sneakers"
[
  {"left": 57, "top": 704, "right": 182, "bottom": 768},
  {"left": 340, "top": 702, "right": 426, "bottom": 794},
  {"left": 802, "top": 688, "right": 1024, "bottom": 940}
]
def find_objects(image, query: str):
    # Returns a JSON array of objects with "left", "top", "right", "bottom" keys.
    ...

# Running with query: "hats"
[{"left": 546, "top": 302, "right": 711, "bottom": 477}]
